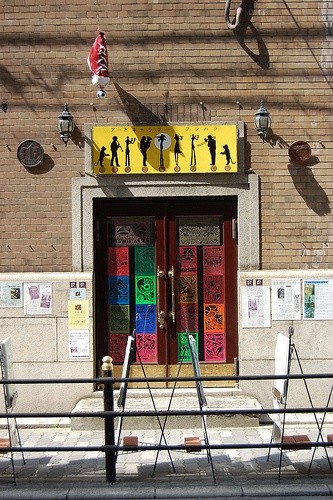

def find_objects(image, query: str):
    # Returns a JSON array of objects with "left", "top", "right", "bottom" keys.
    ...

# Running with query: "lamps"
[
  {"left": 254, "top": 100, "right": 271, "bottom": 142},
  {"left": 56, "top": 102, "right": 76, "bottom": 144}
]
